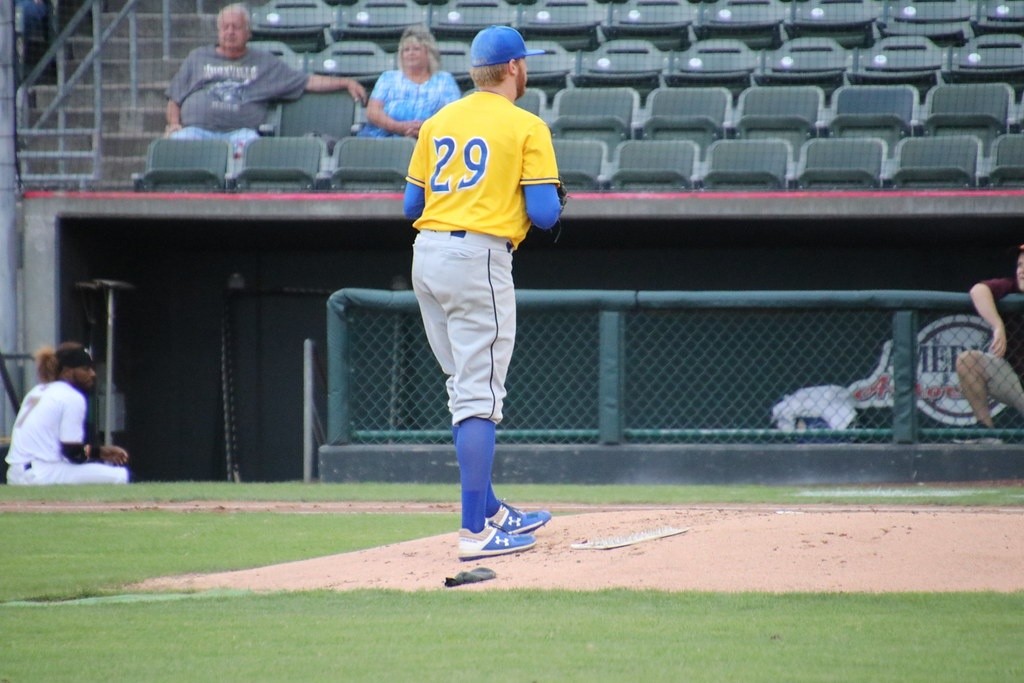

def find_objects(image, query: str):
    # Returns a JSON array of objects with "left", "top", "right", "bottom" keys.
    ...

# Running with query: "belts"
[
  {"left": 451, "top": 230, "right": 512, "bottom": 253},
  {"left": 24, "top": 462, "right": 31, "bottom": 471}
]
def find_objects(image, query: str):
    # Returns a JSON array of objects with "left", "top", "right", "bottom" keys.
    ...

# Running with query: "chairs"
[{"left": 132, "top": 0, "right": 1024, "bottom": 190}]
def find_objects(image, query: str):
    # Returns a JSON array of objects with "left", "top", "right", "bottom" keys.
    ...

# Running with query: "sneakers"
[
  {"left": 950, "top": 421, "right": 1005, "bottom": 445},
  {"left": 487, "top": 498, "right": 552, "bottom": 535},
  {"left": 457, "top": 524, "right": 537, "bottom": 557}
]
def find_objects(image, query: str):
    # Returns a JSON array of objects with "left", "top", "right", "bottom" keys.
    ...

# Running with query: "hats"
[{"left": 470, "top": 25, "right": 545, "bottom": 67}]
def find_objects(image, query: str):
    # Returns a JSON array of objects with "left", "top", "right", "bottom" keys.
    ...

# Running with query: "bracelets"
[{"left": 89, "top": 445, "right": 100, "bottom": 461}]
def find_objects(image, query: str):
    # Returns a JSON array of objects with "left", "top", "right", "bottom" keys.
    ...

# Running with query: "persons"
[
  {"left": 402, "top": 22, "right": 566, "bottom": 563},
  {"left": 4, "top": 342, "right": 130, "bottom": 486},
  {"left": 163, "top": 4, "right": 367, "bottom": 179},
  {"left": 358, "top": 25, "right": 459, "bottom": 137},
  {"left": 955, "top": 243, "right": 1024, "bottom": 443}
]
